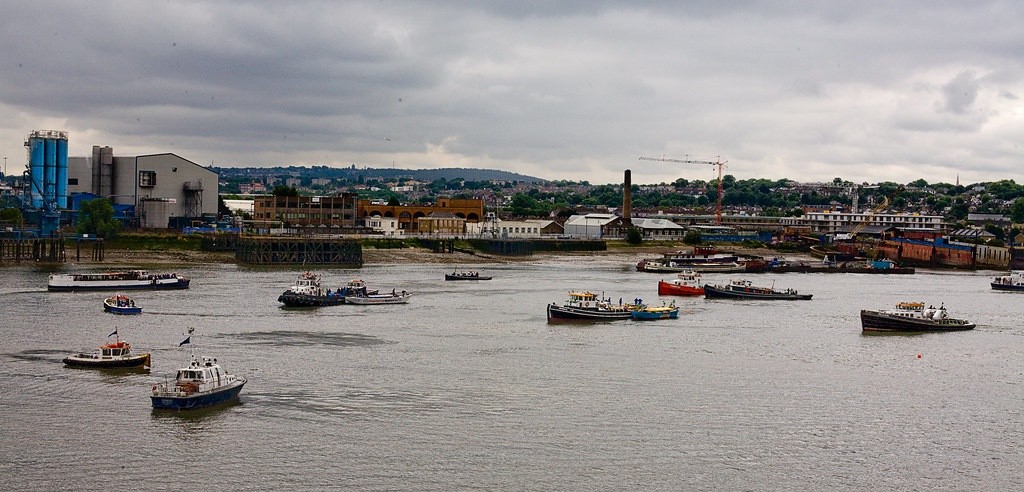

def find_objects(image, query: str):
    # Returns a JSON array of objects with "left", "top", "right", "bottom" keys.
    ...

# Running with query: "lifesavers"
[
  {"left": 684, "top": 282, "right": 687, "bottom": 285},
  {"left": 596, "top": 302, "right": 599, "bottom": 307},
  {"left": 603, "top": 304, "right": 606, "bottom": 309},
  {"left": 585, "top": 302, "right": 589, "bottom": 306}
]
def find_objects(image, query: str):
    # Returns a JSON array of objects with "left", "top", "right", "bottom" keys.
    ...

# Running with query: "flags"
[
  {"left": 108, "top": 330, "right": 117, "bottom": 337},
  {"left": 179, "top": 336, "right": 190, "bottom": 347}
]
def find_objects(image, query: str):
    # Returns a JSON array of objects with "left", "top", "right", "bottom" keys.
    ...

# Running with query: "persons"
[
  {"left": 79, "top": 270, "right": 176, "bottom": 280},
  {"left": 324, "top": 285, "right": 353, "bottom": 299}
]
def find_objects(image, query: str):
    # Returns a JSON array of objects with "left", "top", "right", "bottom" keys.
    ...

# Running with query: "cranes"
[{"left": 638, "top": 153, "right": 728, "bottom": 225}]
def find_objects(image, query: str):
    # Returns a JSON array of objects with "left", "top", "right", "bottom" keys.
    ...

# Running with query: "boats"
[
  {"left": 631, "top": 307, "right": 679, "bottom": 320},
  {"left": 47, "top": 268, "right": 190, "bottom": 292},
  {"left": 346, "top": 285, "right": 412, "bottom": 305},
  {"left": 810, "top": 243, "right": 843, "bottom": 261},
  {"left": 860, "top": 301, "right": 977, "bottom": 334},
  {"left": 149, "top": 327, "right": 248, "bottom": 411},
  {"left": 704, "top": 279, "right": 813, "bottom": 300},
  {"left": 103, "top": 293, "right": 142, "bottom": 314},
  {"left": 445, "top": 265, "right": 493, "bottom": 280},
  {"left": 546, "top": 289, "right": 675, "bottom": 322},
  {"left": 990, "top": 272, "right": 1024, "bottom": 291},
  {"left": 658, "top": 268, "right": 705, "bottom": 297},
  {"left": 62, "top": 325, "right": 150, "bottom": 371},
  {"left": 278, "top": 270, "right": 378, "bottom": 307}
]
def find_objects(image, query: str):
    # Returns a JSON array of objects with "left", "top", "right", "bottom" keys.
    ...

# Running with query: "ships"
[{"left": 635, "top": 239, "right": 775, "bottom": 273}]
